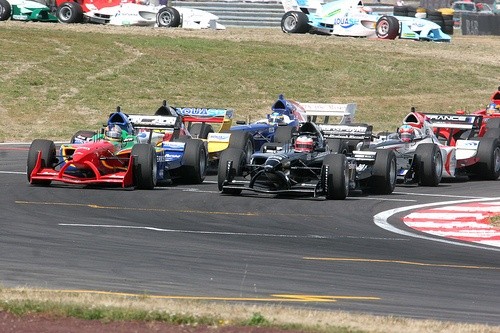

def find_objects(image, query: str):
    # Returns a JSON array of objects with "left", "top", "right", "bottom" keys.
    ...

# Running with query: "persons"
[
  {"left": 104, "top": 125, "right": 123, "bottom": 143},
  {"left": 268, "top": 111, "right": 284, "bottom": 125},
  {"left": 396, "top": 126, "right": 414, "bottom": 140},
  {"left": 486, "top": 104, "right": 500, "bottom": 114},
  {"left": 295, "top": 135, "right": 315, "bottom": 152}
]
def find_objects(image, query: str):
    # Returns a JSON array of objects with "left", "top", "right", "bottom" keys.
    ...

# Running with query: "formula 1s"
[
  {"left": 217, "top": 93, "right": 398, "bottom": 200},
  {"left": 26, "top": 105, "right": 208, "bottom": 190},
  {"left": 137, "top": 100, "right": 247, "bottom": 177},
  {"left": 356, "top": 106, "right": 457, "bottom": 187},
  {"left": 281, "top": 0, "right": 453, "bottom": 41},
  {"left": 55, "top": 0, "right": 227, "bottom": 30},
  {"left": 0, "top": 0, "right": 58, "bottom": 22},
  {"left": 418, "top": 86, "right": 500, "bottom": 181}
]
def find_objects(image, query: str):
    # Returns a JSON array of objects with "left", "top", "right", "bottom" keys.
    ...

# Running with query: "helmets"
[
  {"left": 398, "top": 125, "right": 415, "bottom": 142},
  {"left": 269, "top": 112, "right": 284, "bottom": 125},
  {"left": 104, "top": 125, "right": 122, "bottom": 141},
  {"left": 486, "top": 104, "right": 499, "bottom": 115},
  {"left": 295, "top": 135, "right": 314, "bottom": 153}
]
